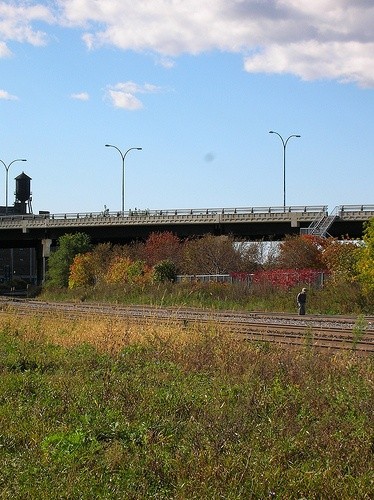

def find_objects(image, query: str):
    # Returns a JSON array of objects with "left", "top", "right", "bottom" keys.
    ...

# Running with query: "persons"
[{"left": 295, "top": 287, "right": 309, "bottom": 315}]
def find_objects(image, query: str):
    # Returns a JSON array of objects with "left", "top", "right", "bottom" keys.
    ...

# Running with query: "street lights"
[
  {"left": 268, "top": 131, "right": 302, "bottom": 213},
  {"left": 0, "top": 159, "right": 27, "bottom": 216},
  {"left": 105, "top": 144, "right": 142, "bottom": 210}
]
